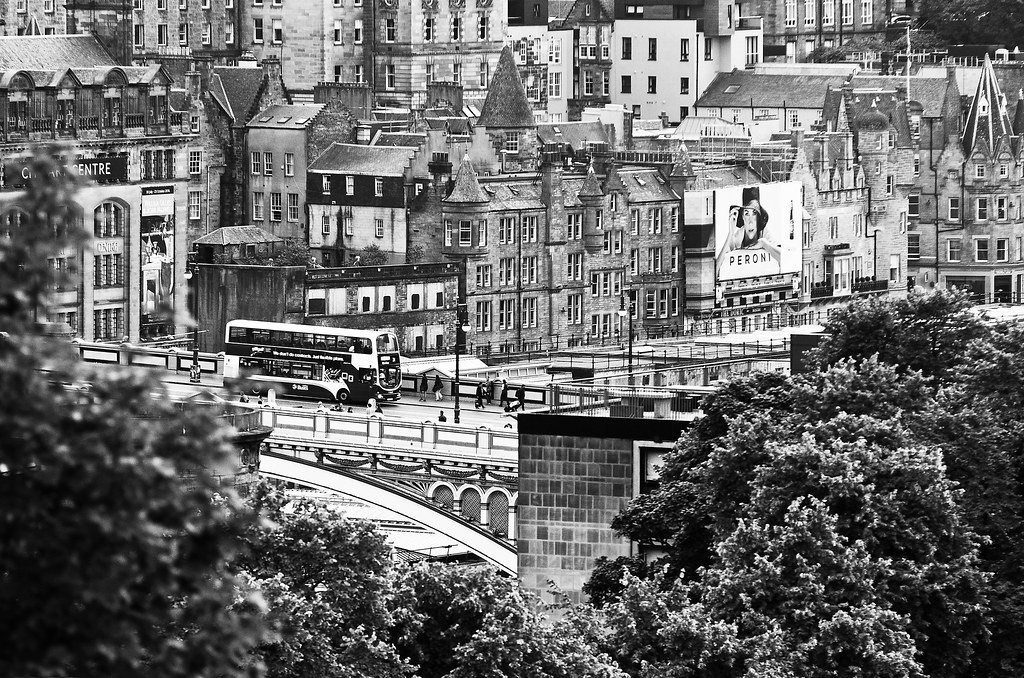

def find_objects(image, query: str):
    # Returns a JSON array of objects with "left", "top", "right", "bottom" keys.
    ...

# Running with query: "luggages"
[{"left": 508, "top": 399, "right": 521, "bottom": 411}]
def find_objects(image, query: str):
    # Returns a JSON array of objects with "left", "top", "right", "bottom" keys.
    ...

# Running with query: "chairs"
[{"left": 256, "top": 337, "right": 327, "bottom": 351}]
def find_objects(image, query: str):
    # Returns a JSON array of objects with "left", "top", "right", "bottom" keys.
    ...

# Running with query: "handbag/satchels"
[
  {"left": 437, "top": 382, "right": 444, "bottom": 388},
  {"left": 475, "top": 399, "right": 481, "bottom": 407}
]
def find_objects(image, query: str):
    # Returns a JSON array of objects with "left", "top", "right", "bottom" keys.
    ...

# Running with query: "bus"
[{"left": 222, "top": 319, "right": 402, "bottom": 405}]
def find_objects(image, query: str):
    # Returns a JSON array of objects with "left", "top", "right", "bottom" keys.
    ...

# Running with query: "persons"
[
  {"left": 475, "top": 379, "right": 495, "bottom": 408},
  {"left": 419, "top": 373, "right": 428, "bottom": 401},
  {"left": 330, "top": 403, "right": 353, "bottom": 413},
  {"left": 514, "top": 384, "right": 526, "bottom": 411},
  {"left": 366, "top": 403, "right": 374, "bottom": 413},
  {"left": 715, "top": 186, "right": 782, "bottom": 279},
  {"left": 499, "top": 380, "right": 510, "bottom": 407},
  {"left": 439, "top": 411, "right": 447, "bottom": 422},
  {"left": 318, "top": 402, "right": 324, "bottom": 408},
  {"left": 143, "top": 214, "right": 174, "bottom": 294},
  {"left": 434, "top": 375, "right": 444, "bottom": 401},
  {"left": 258, "top": 393, "right": 263, "bottom": 404},
  {"left": 240, "top": 391, "right": 249, "bottom": 403},
  {"left": 380, "top": 370, "right": 392, "bottom": 387},
  {"left": 375, "top": 404, "right": 383, "bottom": 414}
]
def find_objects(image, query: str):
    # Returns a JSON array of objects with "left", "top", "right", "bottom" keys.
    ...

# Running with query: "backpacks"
[{"left": 516, "top": 389, "right": 521, "bottom": 397}]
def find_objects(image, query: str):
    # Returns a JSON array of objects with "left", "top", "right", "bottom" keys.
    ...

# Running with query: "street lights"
[
  {"left": 454, "top": 303, "right": 471, "bottom": 424},
  {"left": 617, "top": 287, "right": 633, "bottom": 374},
  {"left": 714, "top": 279, "right": 723, "bottom": 336},
  {"left": 183, "top": 256, "right": 201, "bottom": 383},
  {"left": 791, "top": 272, "right": 800, "bottom": 326}
]
